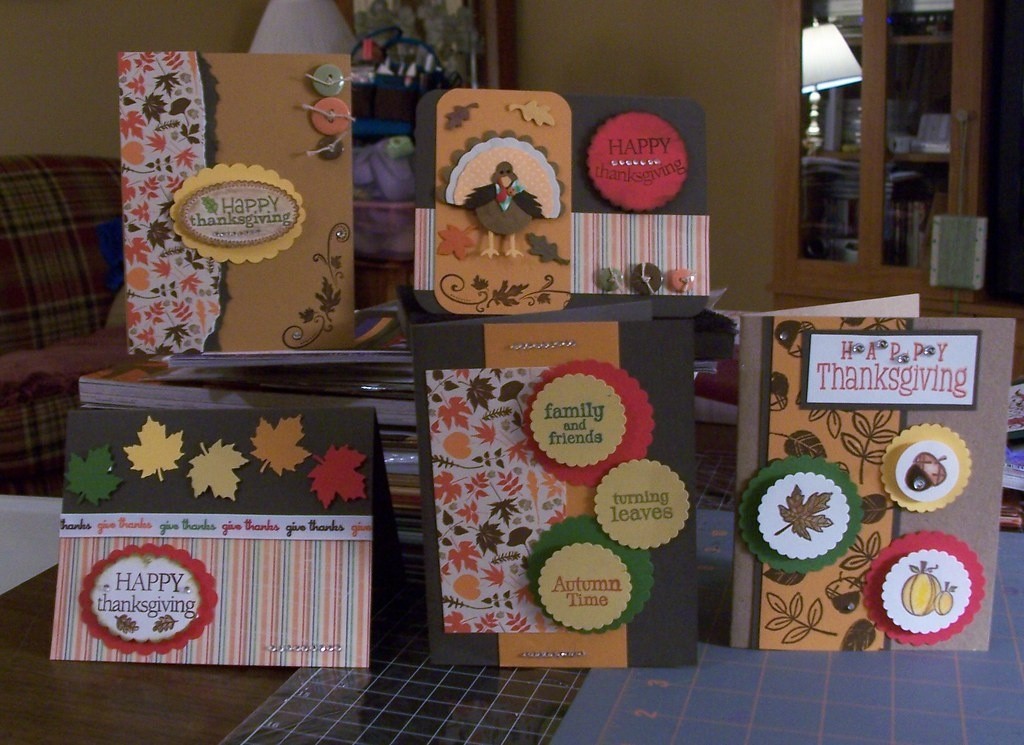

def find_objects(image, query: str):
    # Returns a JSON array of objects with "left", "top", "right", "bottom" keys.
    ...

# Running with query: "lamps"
[{"left": 801, "top": 17, "right": 864, "bottom": 149}]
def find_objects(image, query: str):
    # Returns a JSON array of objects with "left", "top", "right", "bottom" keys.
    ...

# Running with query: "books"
[
  {"left": 804, "top": 0, "right": 954, "bottom": 265},
  {"left": 75, "top": 296, "right": 765, "bottom": 587},
  {"left": 1003, "top": 381, "right": 1023, "bottom": 529}
]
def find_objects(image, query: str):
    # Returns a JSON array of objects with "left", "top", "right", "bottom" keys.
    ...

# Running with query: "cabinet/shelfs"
[{"left": 771, "top": 1, "right": 1005, "bottom": 303}]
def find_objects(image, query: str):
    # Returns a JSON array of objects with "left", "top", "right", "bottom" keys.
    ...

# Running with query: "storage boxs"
[{"left": 353, "top": 200, "right": 416, "bottom": 263}]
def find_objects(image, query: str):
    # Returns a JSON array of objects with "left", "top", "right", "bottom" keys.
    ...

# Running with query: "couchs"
[{"left": 1, "top": 155, "right": 127, "bottom": 479}]
